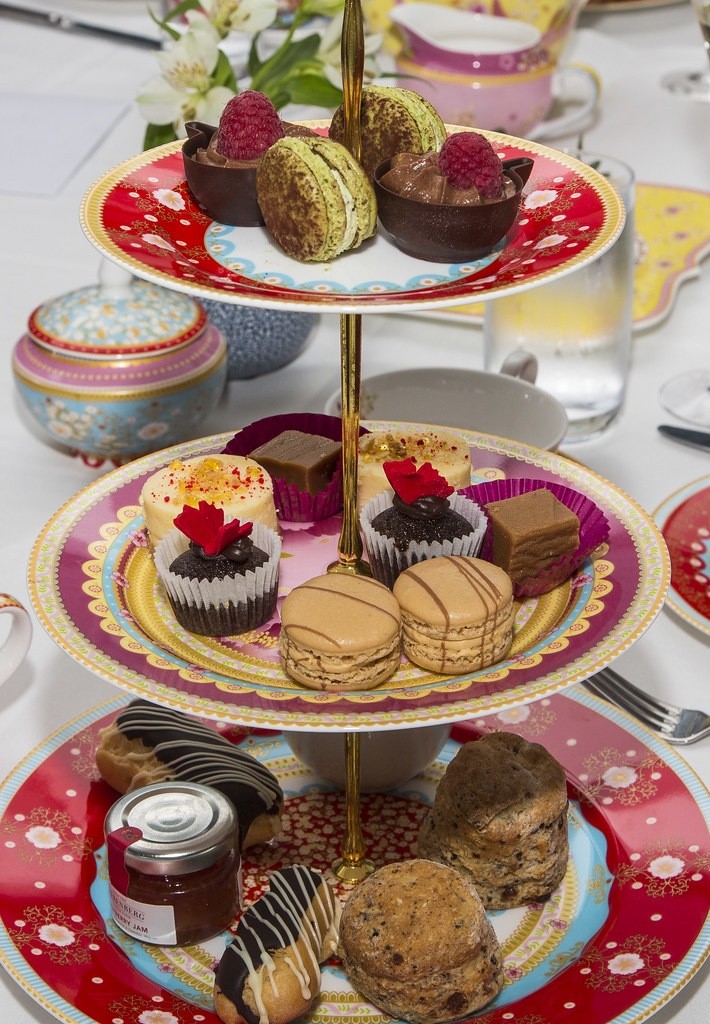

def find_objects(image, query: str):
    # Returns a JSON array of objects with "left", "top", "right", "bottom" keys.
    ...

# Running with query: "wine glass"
[
  {"left": 659, "top": 371, "right": 710, "bottom": 429},
  {"left": 662, "top": 0, "right": 710, "bottom": 102}
]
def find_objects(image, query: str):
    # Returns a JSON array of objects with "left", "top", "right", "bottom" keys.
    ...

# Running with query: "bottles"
[{"left": 104, "top": 781, "right": 241, "bottom": 947}]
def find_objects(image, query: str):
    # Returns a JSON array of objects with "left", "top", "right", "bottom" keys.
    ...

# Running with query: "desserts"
[
  {"left": 183, "top": 84, "right": 536, "bottom": 263},
  {"left": 139, "top": 410, "right": 610, "bottom": 698}
]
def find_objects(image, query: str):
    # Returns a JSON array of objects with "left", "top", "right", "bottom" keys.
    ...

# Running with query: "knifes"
[{"left": 657, "top": 426, "right": 710, "bottom": 452}]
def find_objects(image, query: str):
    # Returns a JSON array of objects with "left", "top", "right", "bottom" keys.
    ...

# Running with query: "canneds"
[{"left": 103, "top": 780, "right": 244, "bottom": 948}]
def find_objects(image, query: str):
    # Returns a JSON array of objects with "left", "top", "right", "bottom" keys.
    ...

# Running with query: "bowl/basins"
[
  {"left": 281, "top": 724, "right": 453, "bottom": 794},
  {"left": 181, "top": 121, "right": 268, "bottom": 227},
  {"left": 131, "top": 272, "right": 320, "bottom": 380},
  {"left": 11, "top": 259, "right": 227, "bottom": 459},
  {"left": 373, "top": 156, "right": 534, "bottom": 264}
]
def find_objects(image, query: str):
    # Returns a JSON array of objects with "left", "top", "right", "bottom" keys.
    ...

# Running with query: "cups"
[
  {"left": 0, "top": 593, "right": 33, "bottom": 688},
  {"left": 392, "top": 3, "right": 602, "bottom": 142},
  {"left": 323, "top": 350, "right": 569, "bottom": 452},
  {"left": 482, "top": 148, "right": 636, "bottom": 448}
]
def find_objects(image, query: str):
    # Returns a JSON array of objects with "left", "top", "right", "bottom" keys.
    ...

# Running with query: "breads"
[{"left": 94, "top": 700, "right": 571, "bottom": 1024}]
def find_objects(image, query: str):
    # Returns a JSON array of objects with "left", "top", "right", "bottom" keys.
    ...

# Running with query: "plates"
[
  {"left": 393, "top": 183, "right": 710, "bottom": 330},
  {"left": 29, "top": 421, "right": 672, "bottom": 732},
  {"left": 584, "top": 0, "right": 687, "bottom": 14},
  {"left": 0, "top": 688, "right": 710, "bottom": 1024},
  {"left": 651, "top": 475, "right": 710, "bottom": 636},
  {"left": 361, "top": 0, "right": 589, "bottom": 57},
  {"left": 80, "top": 118, "right": 626, "bottom": 314}
]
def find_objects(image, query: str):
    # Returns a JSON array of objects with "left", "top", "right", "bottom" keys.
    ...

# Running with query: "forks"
[{"left": 581, "top": 667, "right": 710, "bottom": 745}]
